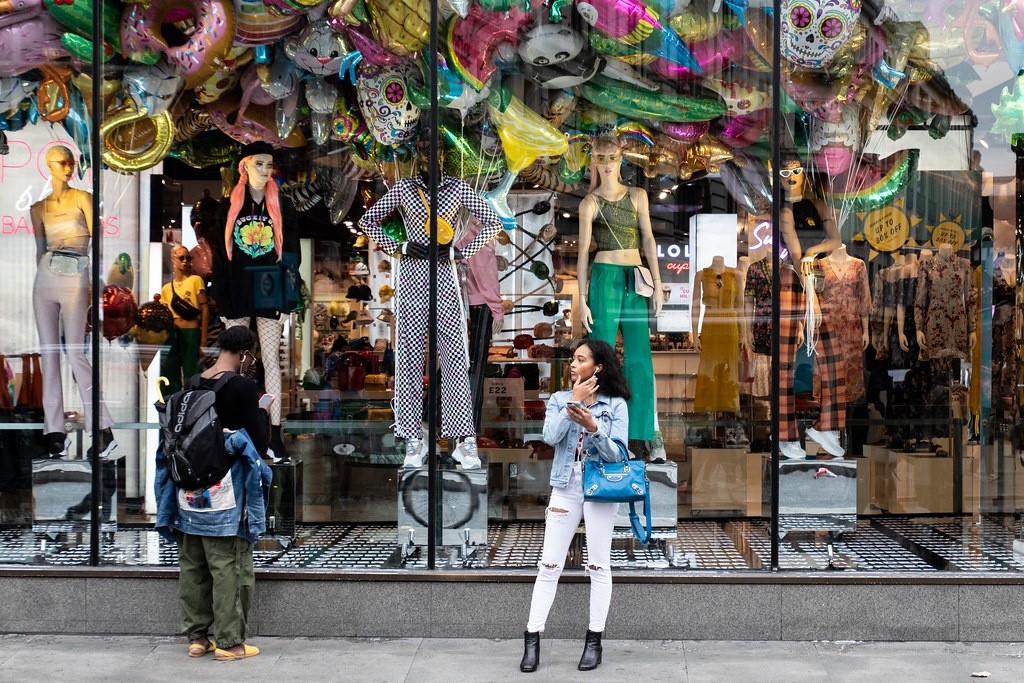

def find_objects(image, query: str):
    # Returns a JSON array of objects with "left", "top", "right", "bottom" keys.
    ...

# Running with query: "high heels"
[
  {"left": 578, "top": 630, "right": 603, "bottom": 670},
  {"left": 519, "top": 631, "right": 541, "bottom": 672}
]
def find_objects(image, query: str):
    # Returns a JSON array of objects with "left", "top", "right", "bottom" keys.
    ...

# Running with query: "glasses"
[
  {"left": 173, "top": 256, "right": 193, "bottom": 262},
  {"left": 241, "top": 350, "right": 256, "bottom": 365}
]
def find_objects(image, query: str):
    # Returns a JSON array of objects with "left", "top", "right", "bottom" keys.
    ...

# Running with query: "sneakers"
[
  {"left": 451, "top": 437, "right": 481, "bottom": 469},
  {"left": 40, "top": 427, "right": 118, "bottom": 458},
  {"left": 265, "top": 424, "right": 291, "bottom": 464},
  {"left": 649, "top": 431, "right": 667, "bottom": 464},
  {"left": 403, "top": 438, "right": 429, "bottom": 468}
]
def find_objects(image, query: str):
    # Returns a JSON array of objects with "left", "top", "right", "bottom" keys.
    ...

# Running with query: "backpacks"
[{"left": 159, "top": 369, "right": 239, "bottom": 490}]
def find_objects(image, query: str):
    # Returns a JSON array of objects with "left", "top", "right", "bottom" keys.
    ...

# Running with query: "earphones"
[
  {"left": 241, "top": 355, "right": 246, "bottom": 362},
  {"left": 594, "top": 367, "right": 600, "bottom": 374}
]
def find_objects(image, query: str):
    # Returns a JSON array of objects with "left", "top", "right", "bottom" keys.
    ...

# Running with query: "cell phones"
[
  {"left": 259, "top": 393, "right": 276, "bottom": 407},
  {"left": 566, "top": 401, "right": 581, "bottom": 414}
]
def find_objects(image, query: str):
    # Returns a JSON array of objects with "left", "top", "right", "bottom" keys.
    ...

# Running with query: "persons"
[
  {"left": 170, "top": 323, "right": 272, "bottom": 660},
  {"left": 28, "top": 141, "right": 367, "bottom": 459},
  {"left": 357, "top": 128, "right": 503, "bottom": 472},
  {"left": 578, "top": 133, "right": 667, "bottom": 460},
  {"left": 521, "top": 339, "right": 631, "bottom": 673},
  {"left": 691, "top": 149, "right": 1021, "bottom": 459},
  {"left": 484, "top": 397, "right": 518, "bottom": 444},
  {"left": 503, "top": 362, "right": 541, "bottom": 388}
]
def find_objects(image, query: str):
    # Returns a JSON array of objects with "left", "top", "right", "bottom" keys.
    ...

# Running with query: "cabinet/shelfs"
[
  {"left": 274, "top": 198, "right": 772, "bottom": 523},
  {"left": 0, "top": 345, "right": 170, "bottom": 526}
]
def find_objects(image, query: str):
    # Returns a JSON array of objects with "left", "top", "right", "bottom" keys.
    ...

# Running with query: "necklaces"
[{"left": 832, "top": 253, "right": 849, "bottom": 273}]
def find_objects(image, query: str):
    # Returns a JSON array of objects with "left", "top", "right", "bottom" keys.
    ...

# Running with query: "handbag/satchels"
[
  {"left": 417, "top": 186, "right": 454, "bottom": 244},
  {"left": 582, "top": 439, "right": 647, "bottom": 503},
  {"left": 286, "top": 353, "right": 556, "bottom": 459},
  {"left": 171, "top": 280, "right": 202, "bottom": 321},
  {"left": 45, "top": 250, "right": 90, "bottom": 276},
  {"left": 633, "top": 265, "right": 654, "bottom": 297}
]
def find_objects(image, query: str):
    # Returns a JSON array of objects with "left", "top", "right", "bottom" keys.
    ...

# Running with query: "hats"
[
  {"left": 329, "top": 198, "right": 572, "bottom": 358},
  {"left": 241, "top": 141, "right": 274, "bottom": 159}
]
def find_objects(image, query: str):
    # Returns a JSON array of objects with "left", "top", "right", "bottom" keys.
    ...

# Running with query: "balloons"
[{"left": 0, "top": 0, "right": 1023, "bottom": 193}]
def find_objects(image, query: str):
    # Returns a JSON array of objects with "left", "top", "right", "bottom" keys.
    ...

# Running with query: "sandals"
[
  {"left": 212, "top": 643, "right": 259, "bottom": 660},
  {"left": 188, "top": 639, "right": 216, "bottom": 658}
]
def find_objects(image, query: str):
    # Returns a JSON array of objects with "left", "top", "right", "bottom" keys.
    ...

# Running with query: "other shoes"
[
  {"left": 724, "top": 423, "right": 981, "bottom": 460},
  {"left": 64, "top": 513, "right": 86, "bottom": 527},
  {"left": 101, "top": 520, "right": 117, "bottom": 528},
  {"left": 627, "top": 449, "right": 636, "bottom": 458}
]
